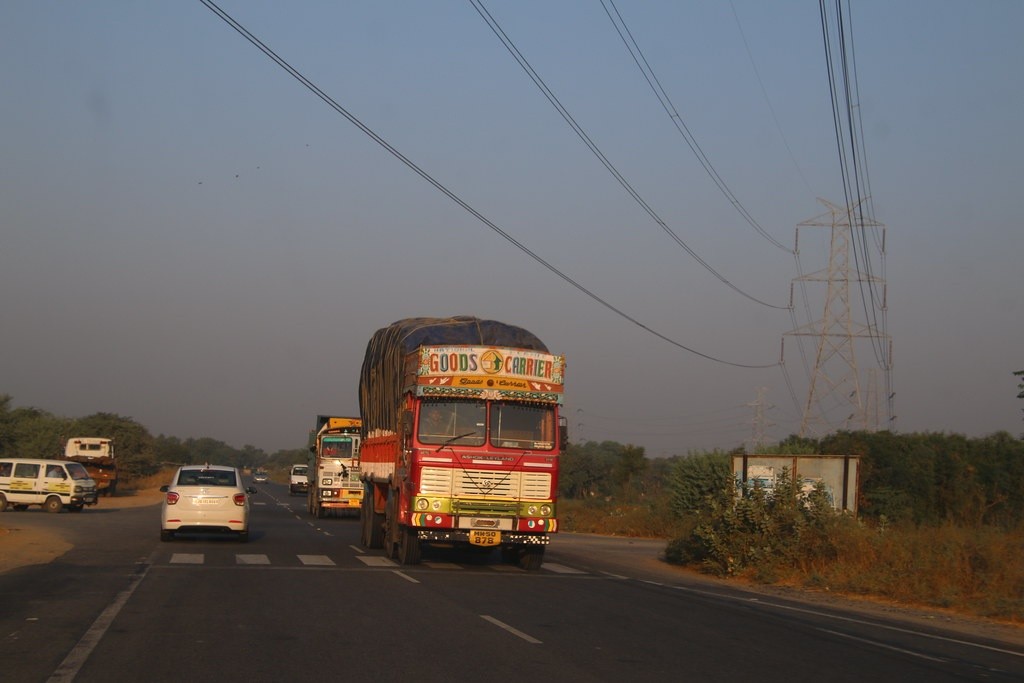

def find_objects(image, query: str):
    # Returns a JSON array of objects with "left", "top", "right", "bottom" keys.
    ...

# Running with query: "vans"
[
  {"left": 0, "top": 457, "right": 99, "bottom": 513},
  {"left": 289, "top": 464, "right": 309, "bottom": 496}
]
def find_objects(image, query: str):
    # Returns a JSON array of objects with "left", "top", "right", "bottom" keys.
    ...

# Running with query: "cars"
[
  {"left": 63, "top": 437, "right": 117, "bottom": 497},
  {"left": 253, "top": 471, "right": 268, "bottom": 484},
  {"left": 158, "top": 462, "right": 257, "bottom": 542}
]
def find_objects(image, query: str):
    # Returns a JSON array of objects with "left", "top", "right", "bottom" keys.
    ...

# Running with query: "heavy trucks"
[
  {"left": 309, "top": 415, "right": 365, "bottom": 519},
  {"left": 353, "top": 313, "right": 569, "bottom": 572}
]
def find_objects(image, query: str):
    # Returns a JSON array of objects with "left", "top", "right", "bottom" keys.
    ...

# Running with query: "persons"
[
  {"left": 421, "top": 407, "right": 451, "bottom": 435},
  {"left": 47, "top": 466, "right": 63, "bottom": 478}
]
